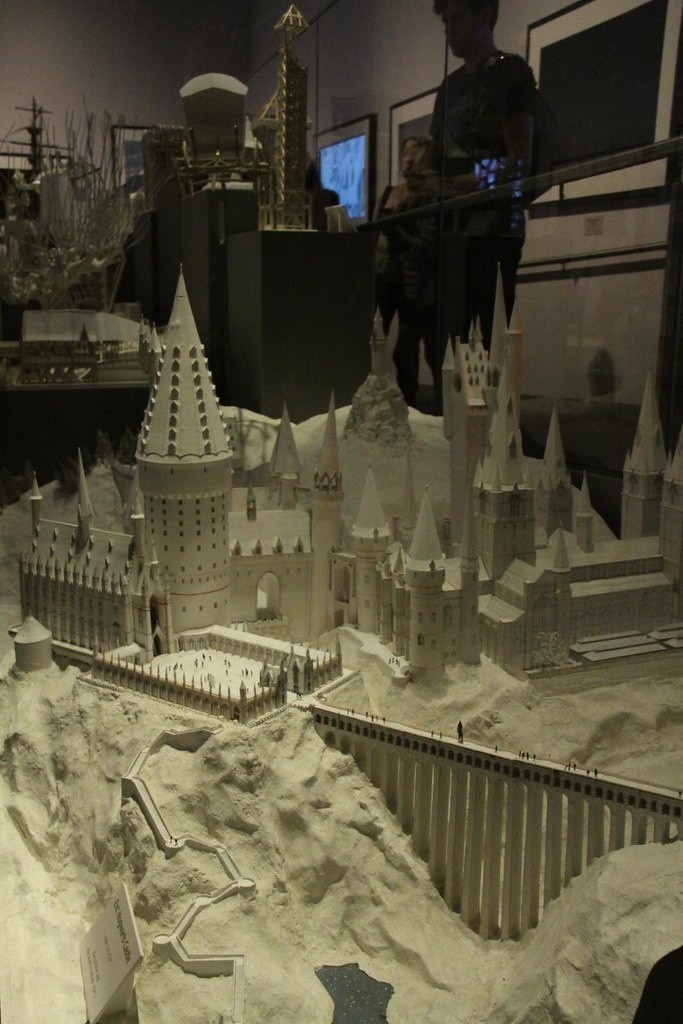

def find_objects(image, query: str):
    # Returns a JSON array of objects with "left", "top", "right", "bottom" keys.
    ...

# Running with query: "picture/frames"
[
  {"left": 312, "top": 112, "right": 378, "bottom": 226},
  {"left": 109, "top": 123, "right": 153, "bottom": 194},
  {"left": 522, "top": 0, "right": 683, "bottom": 192},
  {"left": 388, "top": 85, "right": 442, "bottom": 189}
]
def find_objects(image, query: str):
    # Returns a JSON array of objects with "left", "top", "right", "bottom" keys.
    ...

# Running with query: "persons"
[{"left": 375, "top": 0, "right": 563, "bottom": 417}]
